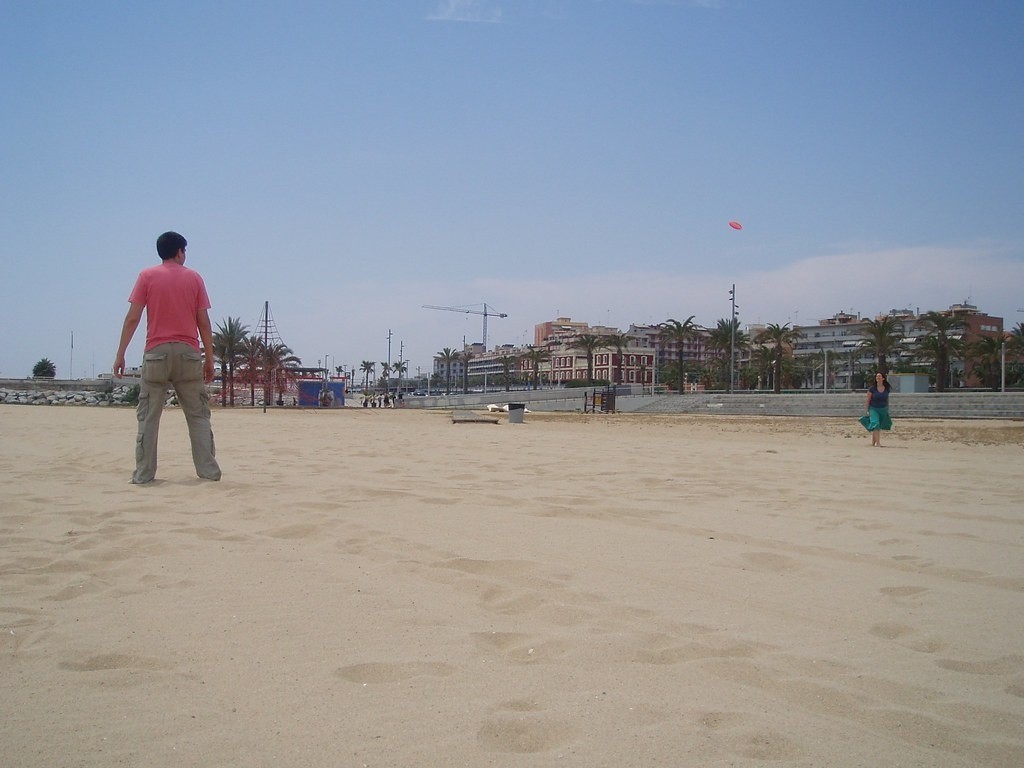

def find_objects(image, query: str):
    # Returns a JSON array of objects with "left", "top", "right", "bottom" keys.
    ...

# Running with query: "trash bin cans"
[{"left": 509, "top": 404, "right": 525, "bottom": 423}]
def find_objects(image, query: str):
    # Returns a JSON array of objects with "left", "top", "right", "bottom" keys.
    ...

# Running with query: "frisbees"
[{"left": 729, "top": 221, "right": 742, "bottom": 230}]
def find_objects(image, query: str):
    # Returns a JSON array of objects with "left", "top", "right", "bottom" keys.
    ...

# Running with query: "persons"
[
  {"left": 858, "top": 372, "right": 892, "bottom": 447},
  {"left": 345, "top": 389, "right": 348, "bottom": 394},
  {"left": 114, "top": 232, "right": 221, "bottom": 484},
  {"left": 360, "top": 392, "right": 403, "bottom": 407}
]
